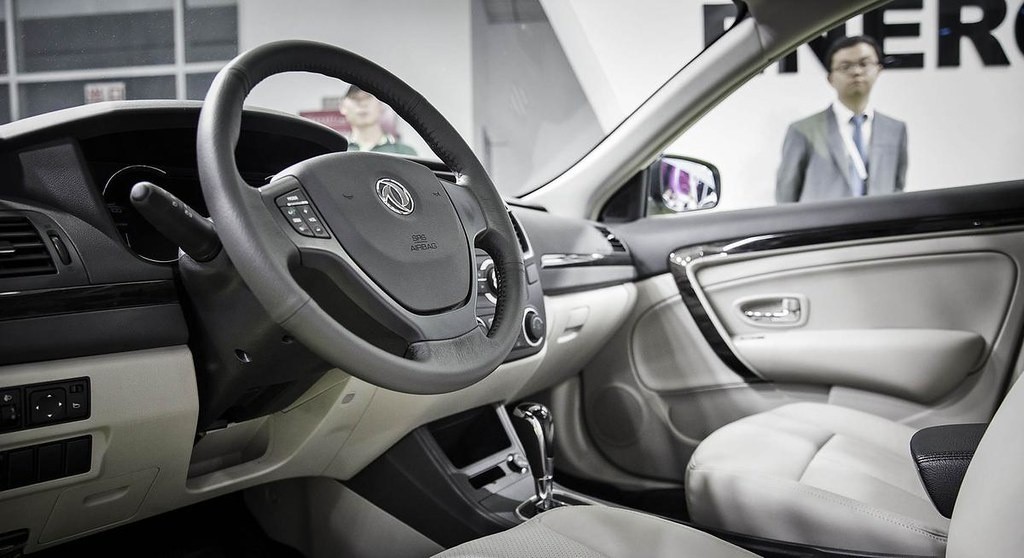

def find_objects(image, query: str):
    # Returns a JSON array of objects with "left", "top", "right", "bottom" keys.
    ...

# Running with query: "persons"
[
  {"left": 776, "top": 36, "right": 908, "bottom": 203},
  {"left": 338, "top": 83, "right": 418, "bottom": 155}
]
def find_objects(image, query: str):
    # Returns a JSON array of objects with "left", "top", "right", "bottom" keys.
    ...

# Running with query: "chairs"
[{"left": 426, "top": 368, "right": 1024, "bottom": 558}]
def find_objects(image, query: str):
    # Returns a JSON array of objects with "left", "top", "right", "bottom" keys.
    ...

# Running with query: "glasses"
[{"left": 832, "top": 58, "right": 879, "bottom": 74}]
